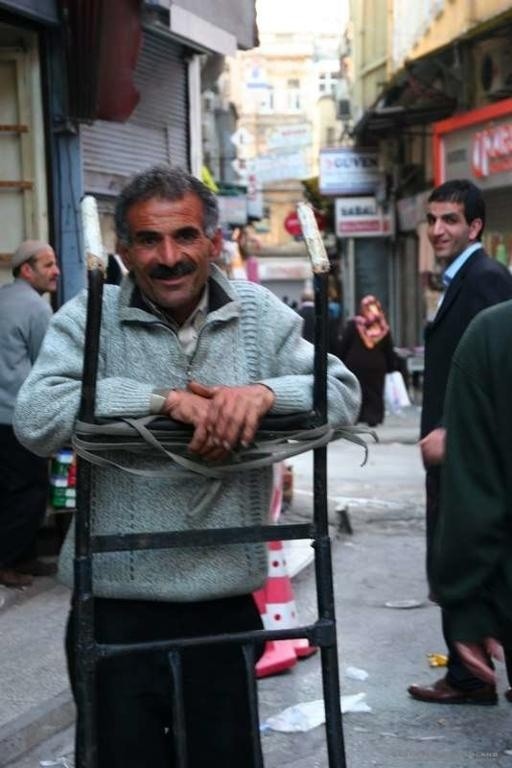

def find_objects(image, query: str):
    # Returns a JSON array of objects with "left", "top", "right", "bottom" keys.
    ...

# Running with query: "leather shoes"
[{"left": 406, "top": 678, "right": 497, "bottom": 705}]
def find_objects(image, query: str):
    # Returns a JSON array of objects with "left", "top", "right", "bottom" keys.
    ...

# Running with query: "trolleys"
[{"left": 60, "top": 193, "right": 349, "bottom": 768}]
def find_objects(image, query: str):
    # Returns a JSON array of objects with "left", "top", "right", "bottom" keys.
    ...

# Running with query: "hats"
[{"left": 11, "top": 241, "right": 49, "bottom": 268}]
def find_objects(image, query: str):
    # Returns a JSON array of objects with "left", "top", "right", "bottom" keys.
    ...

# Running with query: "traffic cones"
[
  {"left": 262, "top": 510, "right": 320, "bottom": 663},
  {"left": 239, "top": 572, "right": 300, "bottom": 680}
]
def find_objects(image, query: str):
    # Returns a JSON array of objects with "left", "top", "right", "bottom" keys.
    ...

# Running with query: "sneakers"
[
  {"left": 18, "top": 562, "right": 57, "bottom": 577},
  {"left": 0, "top": 570, "right": 31, "bottom": 587}
]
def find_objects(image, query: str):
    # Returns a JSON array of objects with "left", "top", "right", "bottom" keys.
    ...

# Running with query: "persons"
[
  {"left": 11, "top": 164, "right": 362, "bottom": 768},
  {"left": 404, "top": 176, "right": 510, "bottom": 707},
  {"left": 426, "top": 301, "right": 509, "bottom": 751},
  {"left": 282, "top": 282, "right": 401, "bottom": 426},
  {"left": 1, "top": 238, "right": 64, "bottom": 586}
]
